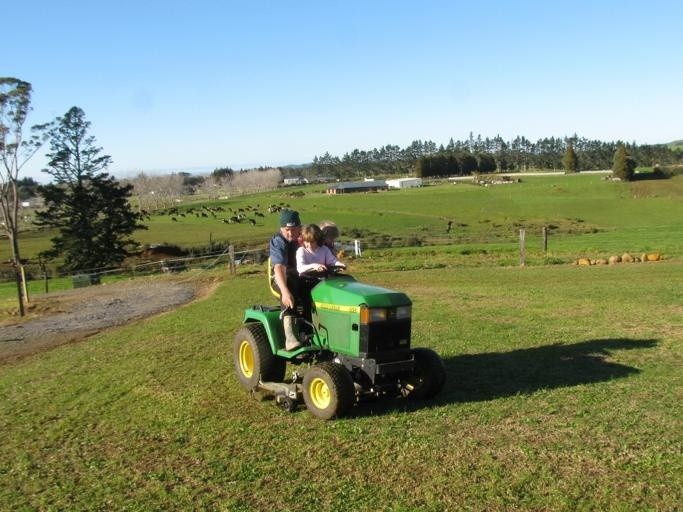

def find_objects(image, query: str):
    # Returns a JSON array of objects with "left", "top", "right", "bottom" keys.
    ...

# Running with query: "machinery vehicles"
[{"left": 235, "top": 257, "right": 447, "bottom": 419}]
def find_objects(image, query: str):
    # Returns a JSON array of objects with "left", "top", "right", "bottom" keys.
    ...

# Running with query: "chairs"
[{"left": 268, "top": 256, "right": 304, "bottom": 306}]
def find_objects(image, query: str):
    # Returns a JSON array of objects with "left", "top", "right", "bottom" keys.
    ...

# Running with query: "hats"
[{"left": 280, "top": 209, "right": 301, "bottom": 227}]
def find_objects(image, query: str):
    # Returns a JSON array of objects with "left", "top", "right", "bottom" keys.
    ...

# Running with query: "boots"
[{"left": 284, "top": 316, "right": 302, "bottom": 351}]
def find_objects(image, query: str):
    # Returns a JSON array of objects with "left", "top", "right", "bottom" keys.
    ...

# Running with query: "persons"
[{"left": 270, "top": 208, "right": 347, "bottom": 351}]
